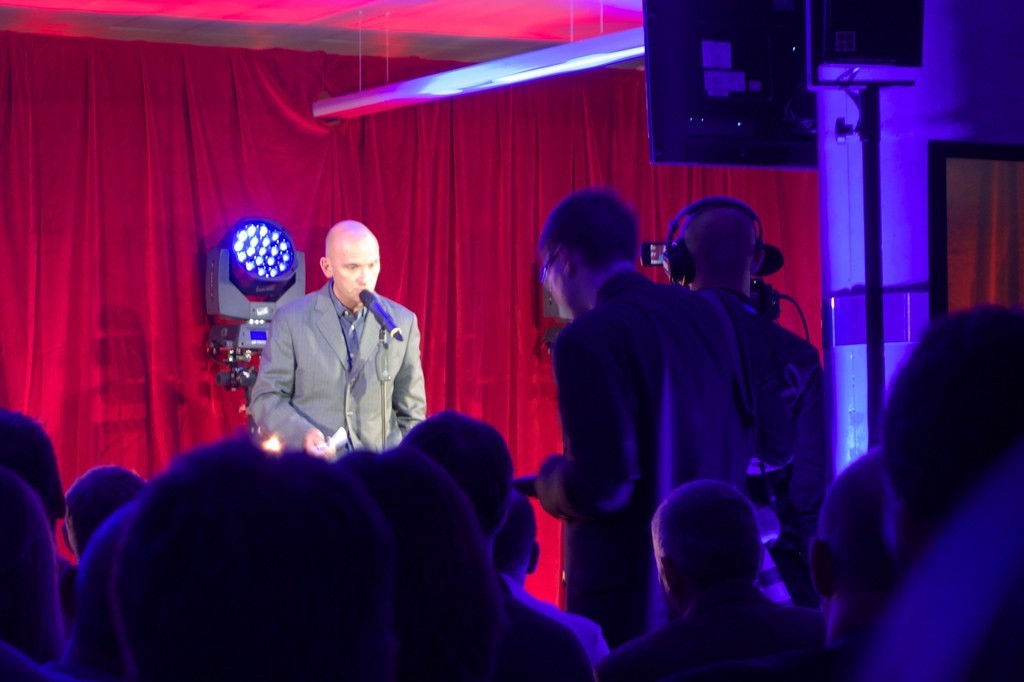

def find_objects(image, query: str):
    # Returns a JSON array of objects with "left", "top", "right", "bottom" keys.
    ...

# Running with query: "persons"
[
  {"left": 534, "top": 189, "right": 757, "bottom": 651},
  {"left": 249, "top": 220, "right": 426, "bottom": 461},
  {"left": 678, "top": 207, "right": 831, "bottom": 608},
  {"left": 0, "top": 303, "right": 1024, "bottom": 682}
]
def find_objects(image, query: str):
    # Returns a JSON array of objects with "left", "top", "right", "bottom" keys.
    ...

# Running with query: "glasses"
[{"left": 542, "top": 243, "right": 563, "bottom": 290}]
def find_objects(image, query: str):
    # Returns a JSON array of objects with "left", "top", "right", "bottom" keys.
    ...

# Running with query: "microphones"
[
  {"left": 359, "top": 289, "right": 404, "bottom": 341},
  {"left": 663, "top": 196, "right": 784, "bottom": 288}
]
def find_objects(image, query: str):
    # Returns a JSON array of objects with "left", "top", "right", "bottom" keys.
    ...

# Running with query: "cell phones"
[{"left": 641, "top": 242, "right": 667, "bottom": 266}]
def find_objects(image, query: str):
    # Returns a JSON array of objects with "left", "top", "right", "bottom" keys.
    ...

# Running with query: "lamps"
[
  {"left": 205, "top": 217, "right": 305, "bottom": 391},
  {"left": 311, "top": 27, "right": 646, "bottom": 117}
]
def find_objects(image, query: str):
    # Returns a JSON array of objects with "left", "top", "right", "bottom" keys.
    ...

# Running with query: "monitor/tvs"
[{"left": 643, "top": 0, "right": 819, "bottom": 170}]
